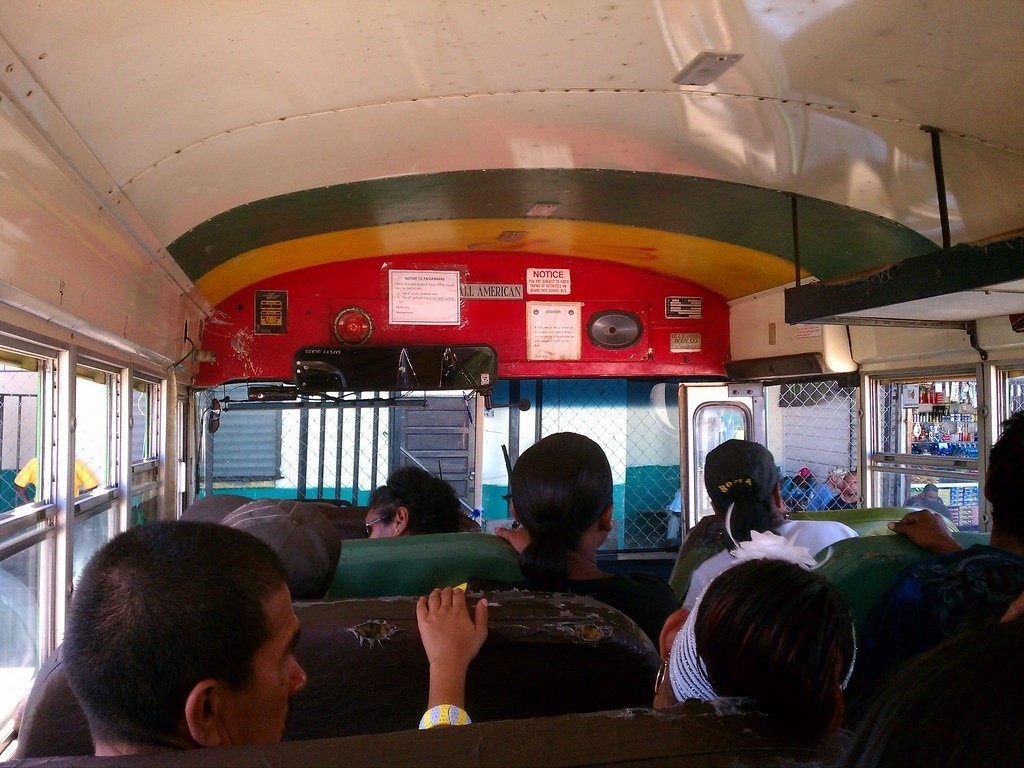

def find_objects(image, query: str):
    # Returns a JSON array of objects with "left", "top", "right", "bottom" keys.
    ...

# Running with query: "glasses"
[{"left": 361, "top": 514, "right": 392, "bottom": 538}]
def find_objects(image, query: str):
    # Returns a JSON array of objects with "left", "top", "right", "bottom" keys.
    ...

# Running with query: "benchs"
[
  {"left": 810, "top": 531, "right": 990, "bottom": 625},
  {"left": 0, "top": 696, "right": 854, "bottom": 767},
  {"left": 323, "top": 532, "right": 520, "bottom": 598},
  {"left": 670, "top": 506, "right": 956, "bottom": 608},
  {"left": 16, "top": 592, "right": 660, "bottom": 756}
]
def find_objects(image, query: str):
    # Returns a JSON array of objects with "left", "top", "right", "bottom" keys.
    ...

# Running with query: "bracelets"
[{"left": 418, "top": 704, "right": 472, "bottom": 730}]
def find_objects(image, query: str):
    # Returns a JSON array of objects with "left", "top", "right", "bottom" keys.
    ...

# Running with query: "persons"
[
  {"left": 824, "top": 471, "right": 858, "bottom": 510},
  {"left": 683, "top": 439, "right": 859, "bottom": 611},
  {"left": 64, "top": 520, "right": 308, "bottom": 757},
  {"left": 833, "top": 622, "right": 1024, "bottom": 768},
  {"left": 774, "top": 466, "right": 842, "bottom": 511},
  {"left": 180, "top": 495, "right": 344, "bottom": 601},
  {"left": 904, "top": 483, "right": 944, "bottom": 508},
  {"left": 14, "top": 454, "right": 99, "bottom": 504},
  {"left": 414, "top": 536, "right": 859, "bottom": 745},
  {"left": 362, "top": 468, "right": 460, "bottom": 539},
  {"left": 490, "top": 432, "right": 681, "bottom": 645},
  {"left": 841, "top": 411, "right": 1024, "bottom": 722}
]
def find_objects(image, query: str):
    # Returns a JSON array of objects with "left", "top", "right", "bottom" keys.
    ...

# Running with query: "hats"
[
  {"left": 180, "top": 494, "right": 342, "bottom": 600},
  {"left": 828, "top": 466, "right": 849, "bottom": 476},
  {"left": 799, "top": 468, "right": 814, "bottom": 477},
  {"left": 704, "top": 440, "right": 777, "bottom": 501}
]
